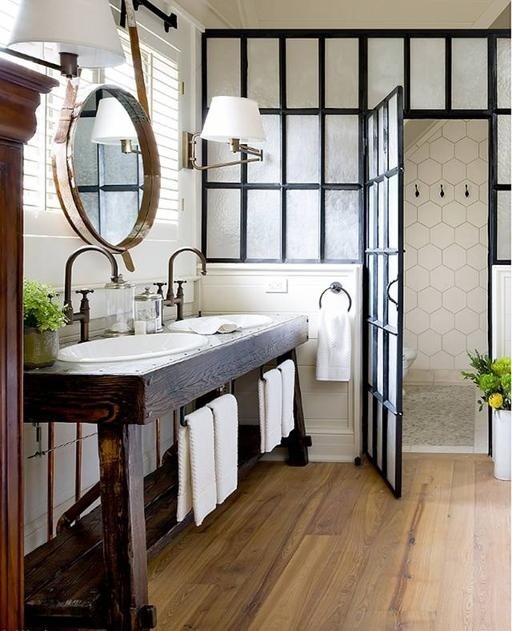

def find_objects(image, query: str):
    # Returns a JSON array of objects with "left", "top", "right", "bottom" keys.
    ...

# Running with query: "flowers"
[{"left": 459, "top": 349, "right": 511, "bottom": 418}]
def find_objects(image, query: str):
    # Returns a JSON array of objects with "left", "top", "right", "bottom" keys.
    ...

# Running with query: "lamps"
[
  {"left": 0, "top": 0, "right": 124, "bottom": 79},
  {"left": 179, "top": 97, "right": 262, "bottom": 171}
]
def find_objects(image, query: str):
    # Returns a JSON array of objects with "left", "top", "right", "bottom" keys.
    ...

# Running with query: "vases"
[{"left": 493, "top": 408, "right": 511, "bottom": 482}]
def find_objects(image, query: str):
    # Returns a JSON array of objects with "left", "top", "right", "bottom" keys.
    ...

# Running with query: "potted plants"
[{"left": 24, "top": 278, "right": 71, "bottom": 369}]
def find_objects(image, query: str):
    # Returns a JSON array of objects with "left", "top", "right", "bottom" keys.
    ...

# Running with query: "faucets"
[
  {"left": 167, "top": 246, "right": 207, "bottom": 300},
  {"left": 62, "top": 245, "right": 118, "bottom": 316}
]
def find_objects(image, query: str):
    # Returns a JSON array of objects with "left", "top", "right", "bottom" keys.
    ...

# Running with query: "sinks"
[
  {"left": 169, "top": 314, "right": 272, "bottom": 333},
  {"left": 59, "top": 333, "right": 204, "bottom": 361}
]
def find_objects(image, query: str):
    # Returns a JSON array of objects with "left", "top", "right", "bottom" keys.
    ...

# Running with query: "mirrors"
[{"left": 55, "top": 84, "right": 163, "bottom": 252}]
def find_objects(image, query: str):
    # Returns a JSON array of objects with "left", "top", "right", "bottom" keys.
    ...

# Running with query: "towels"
[
  {"left": 189, "top": 315, "right": 244, "bottom": 335},
  {"left": 316, "top": 307, "right": 351, "bottom": 381},
  {"left": 177, "top": 393, "right": 238, "bottom": 528},
  {"left": 259, "top": 359, "right": 295, "bottom": 452}
]
